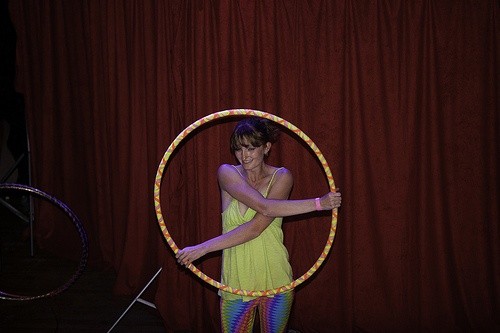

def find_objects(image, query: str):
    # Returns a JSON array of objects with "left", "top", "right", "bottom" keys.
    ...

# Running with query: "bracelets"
[{"left": 315, "top": 198, "right": 322, "bottom": 211}]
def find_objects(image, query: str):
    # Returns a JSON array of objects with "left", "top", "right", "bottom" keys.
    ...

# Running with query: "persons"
[{"left": 174, "top": 119, "right": 343, "bottom": 333}]
